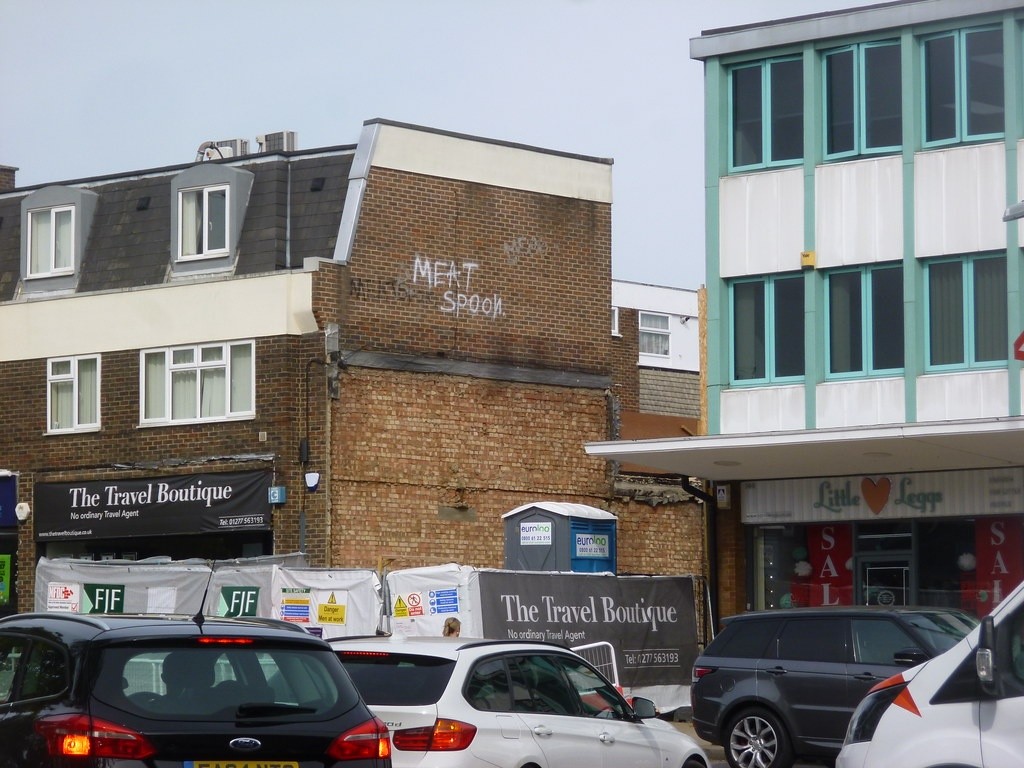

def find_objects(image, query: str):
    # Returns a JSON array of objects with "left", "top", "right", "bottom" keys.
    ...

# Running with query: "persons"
[
  {"left": 442, "top": 617, "right": 461, "bottom": 637},
  {"left": 143, "top": 653, "right": 187, "bottom": 715}
]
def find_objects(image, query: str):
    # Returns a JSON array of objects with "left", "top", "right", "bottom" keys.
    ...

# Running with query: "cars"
[{"left": 832, "top": 582, "right": 1024, "bottom": 768}]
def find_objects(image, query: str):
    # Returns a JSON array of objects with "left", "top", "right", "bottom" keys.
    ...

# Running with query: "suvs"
[
  {"left": 267, "top": 633, "right": 711, "bottom": 768},
  {"left": 0, "top": 610, "right": 393, "bottom": 768},
  {"left": 687, "top": 602, "right": 982, "bottom": 768}
]
